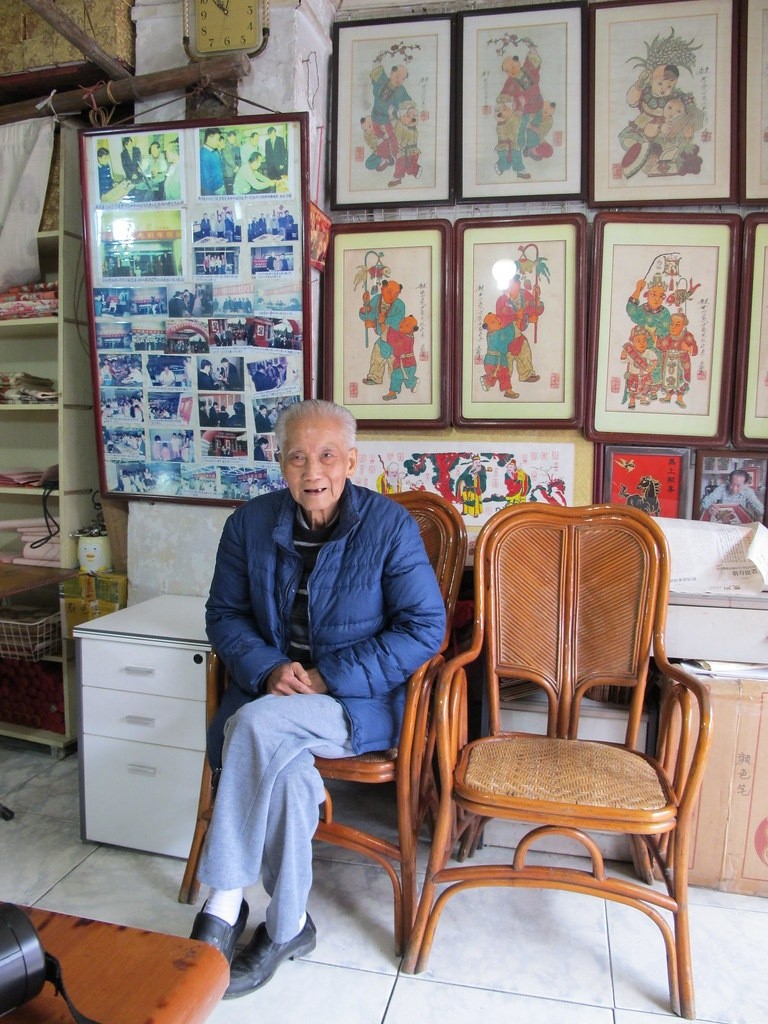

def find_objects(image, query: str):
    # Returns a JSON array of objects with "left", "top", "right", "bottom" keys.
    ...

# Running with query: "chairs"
[
  {"left": 177, "top": 490, "right": 469, "bottom": 958},
  {"left": 400, "top": 503, "right": 713, "bottom": 1020}
]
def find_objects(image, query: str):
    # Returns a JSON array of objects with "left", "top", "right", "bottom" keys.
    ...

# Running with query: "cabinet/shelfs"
[
  {"left": 0, "top": 115, "right": 101, "bottom": 759},
  {"left": 484, "top": 692, "right": 656, "bottom": 864},
  {"left": 72, "top": 595, "right": 211, "bottom": 858}
]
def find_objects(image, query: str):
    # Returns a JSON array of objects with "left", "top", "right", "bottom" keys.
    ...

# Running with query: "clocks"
[{"left": 183, "top": 1, "right": 270, "bottom": 62}]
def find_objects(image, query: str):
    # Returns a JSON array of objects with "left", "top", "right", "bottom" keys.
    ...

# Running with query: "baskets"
[{"left": 0, "top": 608, "right": 61, "bottom": 662}]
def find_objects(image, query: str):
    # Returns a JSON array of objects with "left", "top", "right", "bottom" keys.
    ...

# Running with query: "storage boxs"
[
  {"left": 656, "top": 670, "right": 768, "bottom": 897},
  {"left": 64, "top": 569, "right": 127, "bottom": 640}
]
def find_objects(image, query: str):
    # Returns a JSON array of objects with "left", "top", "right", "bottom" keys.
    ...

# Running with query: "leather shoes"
[
  {"left": 223, "top": 911, "right": 317, "bottom": 998},
  {"left": 189, "top": 898, "right": 249, "bottom": 965}
]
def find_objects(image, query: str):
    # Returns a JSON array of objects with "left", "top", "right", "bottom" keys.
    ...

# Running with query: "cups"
[{"left": 76, "top": 536, "right": 113, "bottom": 572}]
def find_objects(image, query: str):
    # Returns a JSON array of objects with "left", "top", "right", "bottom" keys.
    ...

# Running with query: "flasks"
[{"left": 0, "top": 902, "right": 50, "bottom": 1016}]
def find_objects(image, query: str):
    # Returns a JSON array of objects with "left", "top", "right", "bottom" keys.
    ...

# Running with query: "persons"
[
  {"left": 99, "top": 137, "right": 168, "bottom": 202},
  {"left": 215, "top": 329, "right": 246, "bottom": 347},
  {"left": 100, "top": 358, "right": 193, "bottom": 388},
  {"left": 150, "top": 296, "right": 157, "bottom": 314},
  {"left": 188, "top": 399, "right": 446, "bottom": 999},
  {"left": 201, "top": 127, "right": 286, "bottom": 197},
  {"left": 249, "top": 331, "right": 302, "bottom": 350},
  {"left": 102, "top": 249, "right": 179, "bottom": 276},
  {"left": 255, "top": 298, "right": 302, "bottom": 311},
  {"left": 267, "top": 251, "right": 289, "bottom": 271},
  {"left": 100, "top": 392, "right": 288, "bottom": 499},
  {"left": 101, "top": 293, "right": 117, "bottom": 313},
  {"left": 213, "top": 296, "right": 252, "bottom": 312},
  {"left": 176, "top": 341, "right": 199, "bottom": 352},
  {"left": 168, "top": 289, "right": 210, "bottom": 318},
  {"left": 203, "top": 254, "right": 226, "bottom": 274},
  {"left": 198, "top": 356, "right": 242, "bottom": 391},
  {"left": 700, "top": 471, "right": 764, "bottom": 517},
  {"left": 252, "top": 362, "right": 286, "bottom": 392},
  {"left": 201, "top": 210, "right": 236, "bottom": 242},
  {"left": 136, "top": 337, "right": 166, "bottom": 350},
  {"left": 248, "top": 211, "right": 294, "bottom": 242}
]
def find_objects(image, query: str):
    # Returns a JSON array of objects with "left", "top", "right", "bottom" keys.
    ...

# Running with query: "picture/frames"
[
  {"left": 317, "top": 219, "right": 454, "bottom": 432},
  {"left": 453, "top": 213, "right": 589, "bottom": 430},
  {"left": 328, "top": 12, "right": 455, "bottom": 211},
  {"left": 457, "top": 0, "right": 589, "bottom": 202},
  {"left": 694, "top": 447, "right": 768, "bottom": 528},
  {"left": 79, "top": 112, "right": 313, "bottom": 509},
  {"left": 737, "top": 1, "right": 768, "bottom": 206},
  {"left": 731, "top": 211, "right": 768, "bottom": 452},
  {"left": 350, "top": 428, "right": 603, "bottom": 591},
  {"left": 588, "top": 0, "right": 739, "bottom": 211},
  {"left": 602, "top": 446, "right": 686, "bottom": 518},
  {"left": 585, "top": 210, "right": 744, "bottom": 448}
]
